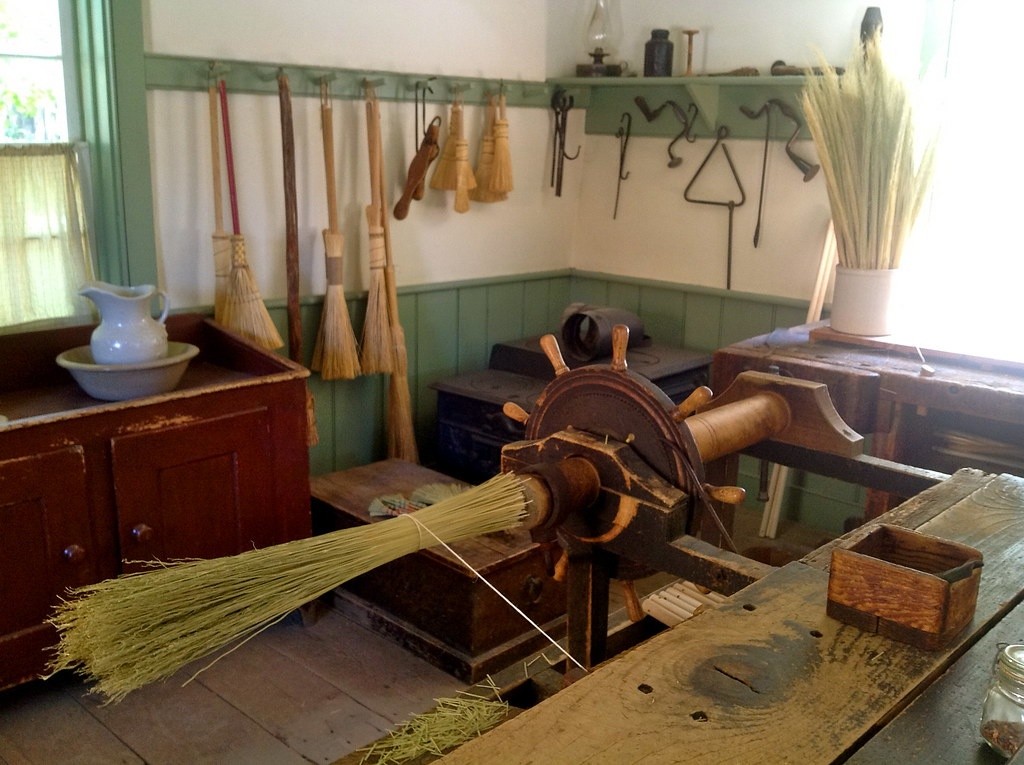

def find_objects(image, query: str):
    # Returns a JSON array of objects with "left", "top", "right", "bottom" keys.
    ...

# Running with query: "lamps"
[{"left": 575, "top": 0, "right": 623, "bottom": 78}]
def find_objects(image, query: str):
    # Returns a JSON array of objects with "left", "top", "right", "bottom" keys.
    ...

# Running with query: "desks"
[
  {"left": 431, "top": 466, "right": 1024, "bottom": 765},
  {"left": 692, "top": 319, "right": 1024, "bottom": 592}
]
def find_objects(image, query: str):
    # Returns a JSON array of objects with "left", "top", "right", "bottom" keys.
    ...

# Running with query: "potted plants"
[{"left": 795, "top": 22, "right": 938, "bottom": 335}]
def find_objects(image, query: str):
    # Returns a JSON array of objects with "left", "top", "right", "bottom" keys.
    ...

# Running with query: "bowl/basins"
[{"left": 56, "top": 342, "right": 199, "bottom": 401}]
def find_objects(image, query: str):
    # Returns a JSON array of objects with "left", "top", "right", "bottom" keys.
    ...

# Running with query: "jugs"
[{"left": 78, "top": 280, "right": 170, "bottom": 367}]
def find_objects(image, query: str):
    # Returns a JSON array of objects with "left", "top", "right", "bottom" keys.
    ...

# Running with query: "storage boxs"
[
  {"left": 311, "top": 455, "right": 568, "bottom": 684},
  {"left": 822, "top": 523, "right": 984, "bottom": 650}
]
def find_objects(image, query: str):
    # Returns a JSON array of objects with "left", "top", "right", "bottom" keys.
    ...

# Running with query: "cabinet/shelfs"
[{"left": 0, "top": 311, "right": 312, "bottom": 702}]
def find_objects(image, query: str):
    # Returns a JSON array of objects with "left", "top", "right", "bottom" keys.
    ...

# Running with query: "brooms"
[
  {"left": 275, "top": 71, "right": 320, "bottom": 448},
  {"left": 357, "top": 99, "right": 421, "bottom": 467},
  {"left": 207, "top": 79, "right": 286, "bottom": 352},
  {"left": 307, "top": 107, "right": 363, "bottom": 383},
  {"left": 428, "top": 93, "right": 514, "bottom": 214}
]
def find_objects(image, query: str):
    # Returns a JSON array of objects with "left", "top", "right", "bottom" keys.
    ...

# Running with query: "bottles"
[{"left": 644, "top": 29, "right": 674, "bottom": 78}]
